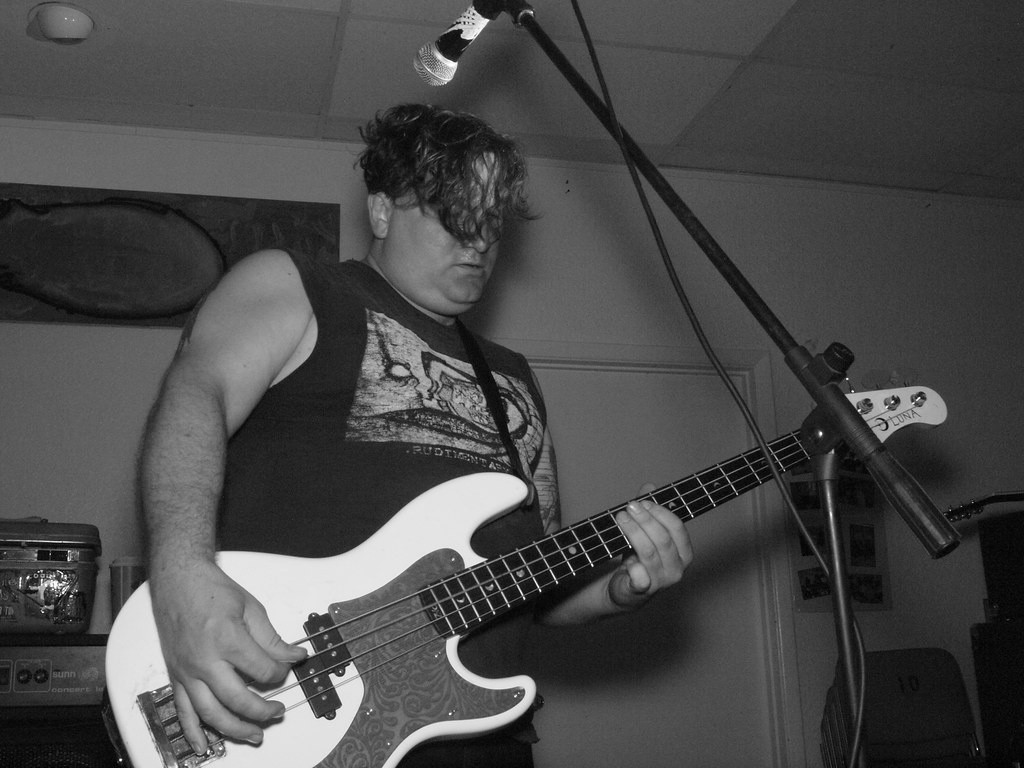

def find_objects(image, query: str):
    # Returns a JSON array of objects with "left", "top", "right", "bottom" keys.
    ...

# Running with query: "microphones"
[{"left": 412, "top": 0, "right": 503, "bottom": 85}]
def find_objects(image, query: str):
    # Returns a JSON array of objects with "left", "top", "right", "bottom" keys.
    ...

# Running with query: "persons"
[{"left": 137, "top": 100, "right": 697, "bottom": 768}]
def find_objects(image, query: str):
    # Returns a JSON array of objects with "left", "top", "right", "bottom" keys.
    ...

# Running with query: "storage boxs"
[{"left": 0, "top": 518, "right": 102, "bottom": 634}]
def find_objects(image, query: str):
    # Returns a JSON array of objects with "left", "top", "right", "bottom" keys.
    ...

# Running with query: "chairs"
[{"left": 820, "top": 648, "right": 981, "bottom": 768}]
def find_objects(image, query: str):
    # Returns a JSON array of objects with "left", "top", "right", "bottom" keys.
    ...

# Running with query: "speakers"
[{"left": 0, "top": 633, "right": 126, "bottom": 768}]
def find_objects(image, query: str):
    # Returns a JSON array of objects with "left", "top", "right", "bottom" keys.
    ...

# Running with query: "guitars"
[{"left": 95, "top": 377, "right": 952, "bottom": 768}]
